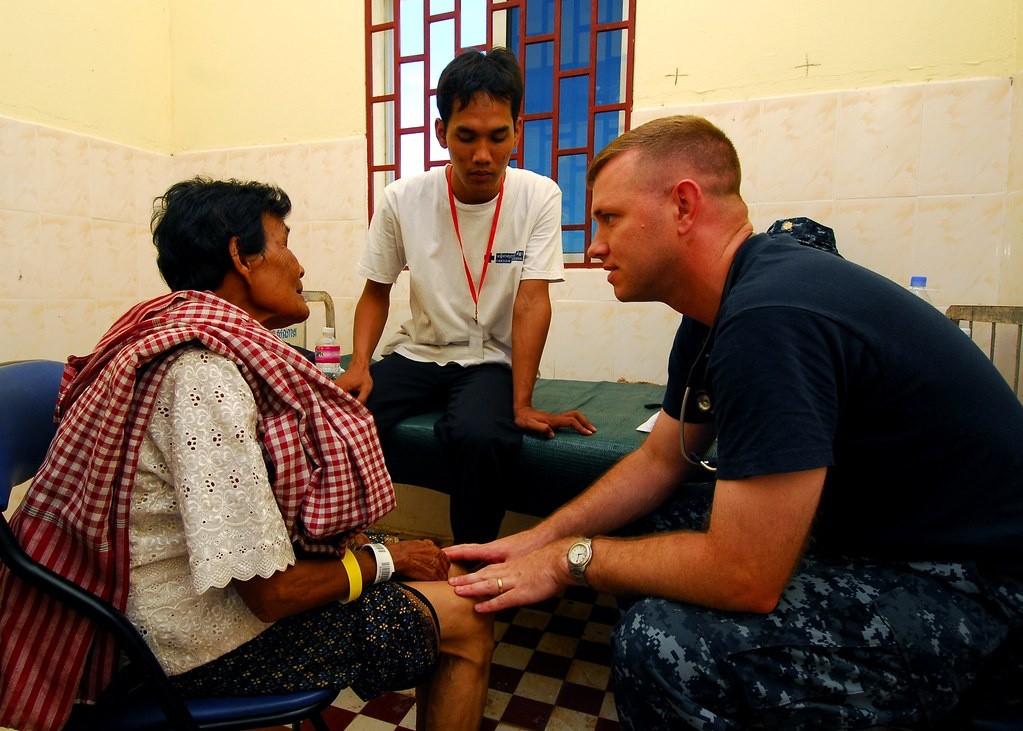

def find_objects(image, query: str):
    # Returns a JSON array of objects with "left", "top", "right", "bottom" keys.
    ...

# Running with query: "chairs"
[
  {"left": 0, "top": 359, "right": 340, "bottom": 731},
  {"left": 945, "top": 305, "right": 1023, "bottom": 405}
]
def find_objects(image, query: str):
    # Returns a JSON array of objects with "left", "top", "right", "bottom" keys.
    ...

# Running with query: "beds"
[{"left": 330, "top": 353, "right": 719, "bottom": 536}]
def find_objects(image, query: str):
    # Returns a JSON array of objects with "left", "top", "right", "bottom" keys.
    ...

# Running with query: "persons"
[
  {"left": 439, "top": 116, "right": 1022, "bottom": 726},
  {"left": 1, "top": 180, "right": 497, "bottom": 731},
  {"left": 333, "top": 46, "right": 597, "bottom": 653}
]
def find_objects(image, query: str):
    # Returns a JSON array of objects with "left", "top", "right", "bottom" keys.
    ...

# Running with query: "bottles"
[{"left": 315, "top": 328, "right": 341, "bottom": 381}]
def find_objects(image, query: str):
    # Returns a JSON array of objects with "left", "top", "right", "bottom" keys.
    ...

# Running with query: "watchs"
[{"left": 566, "top": 537, "right": 593, "bottom": 581}]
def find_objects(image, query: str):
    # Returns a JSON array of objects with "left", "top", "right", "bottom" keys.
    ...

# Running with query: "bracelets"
[{"left": 339, "top": 549, "right": 363, "bottom": 605}]
